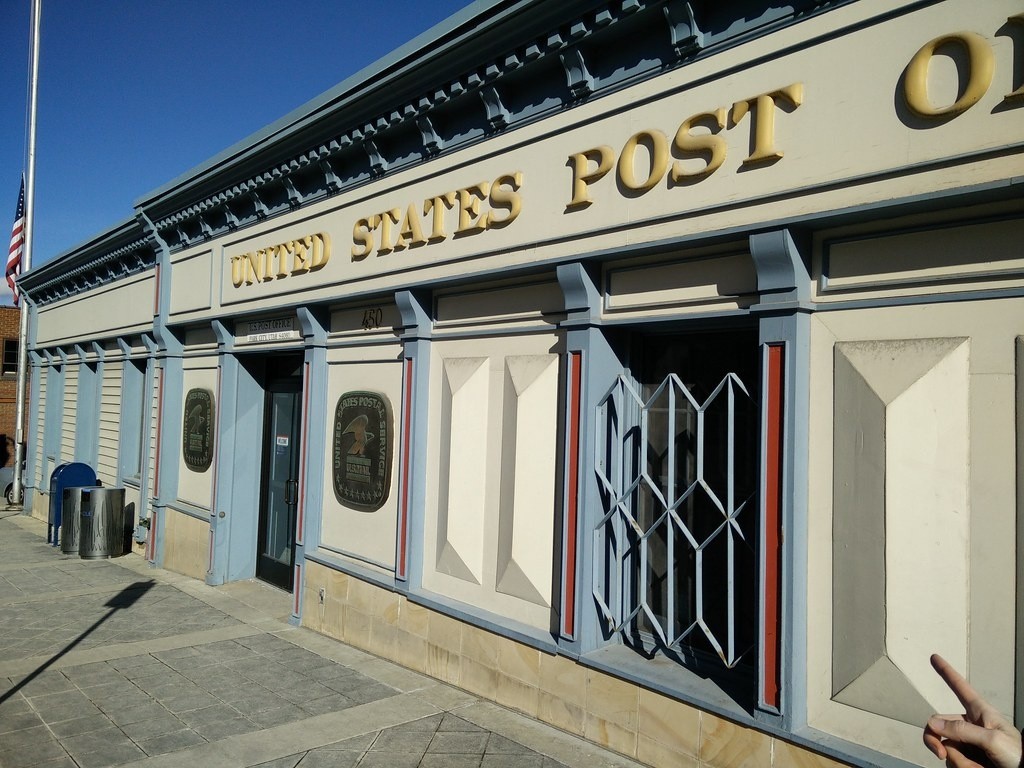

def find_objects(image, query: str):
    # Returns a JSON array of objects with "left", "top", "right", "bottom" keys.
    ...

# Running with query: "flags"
[{"left": 5, "top": 174, "right": 26, "bottom": 308}]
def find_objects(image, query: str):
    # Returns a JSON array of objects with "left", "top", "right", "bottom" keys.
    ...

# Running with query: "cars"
[{"left": 0, "top": 460, "right": 26, "bottom": 505}]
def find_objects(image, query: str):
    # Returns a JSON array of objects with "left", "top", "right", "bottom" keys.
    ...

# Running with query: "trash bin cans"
[
  {"left": 46, "top": 461, "right": 102, "bottom": 547},
  {"left": 60, "top": 485, "right": 105, "bottom": 556},
  {"left": 78, "top": 488, "right": 126, "bottom": 560}
]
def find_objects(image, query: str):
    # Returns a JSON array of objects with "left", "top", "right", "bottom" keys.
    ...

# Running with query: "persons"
[{"left": 922, "top": 653, "right": 1024, "bottom": 768}]
